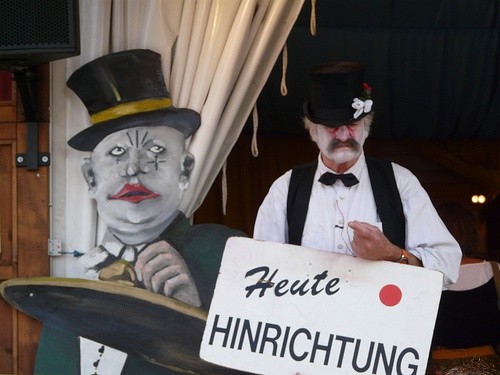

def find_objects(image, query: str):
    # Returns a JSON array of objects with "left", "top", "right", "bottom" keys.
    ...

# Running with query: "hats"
[{"left": 304, "top": 60, "right": 373, "bottom": 127}]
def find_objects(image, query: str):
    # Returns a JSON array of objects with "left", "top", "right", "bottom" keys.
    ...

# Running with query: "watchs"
[{"left": 396, "top": 250, "right": 409, "bottom": 265}]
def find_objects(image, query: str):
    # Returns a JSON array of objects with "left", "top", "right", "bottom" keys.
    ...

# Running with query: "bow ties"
[{"left": 318, "top": 172, "right": 359, "bottom": 187}]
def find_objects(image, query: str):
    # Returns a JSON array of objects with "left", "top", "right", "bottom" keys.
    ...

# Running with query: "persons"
[
  {"left": 250, "top": 64, "right": 463, "bottom": 291},
  {"left": 429, "top": 167, "right": 499, "bottom": 347}
]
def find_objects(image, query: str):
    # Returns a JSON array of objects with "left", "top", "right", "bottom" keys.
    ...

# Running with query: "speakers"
[{"left": 0, "top": 0, "right": 81, "bottom": 68}]
